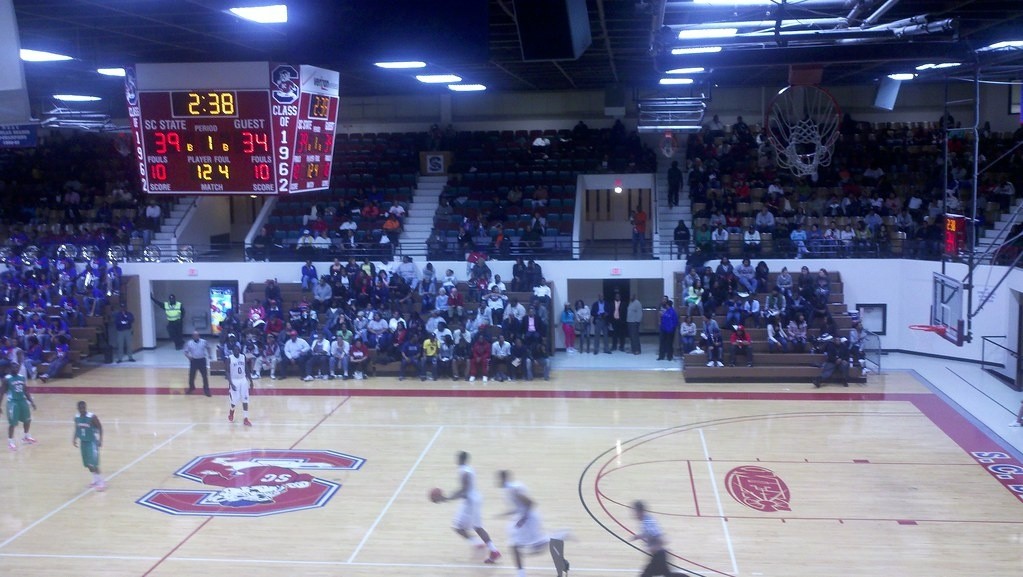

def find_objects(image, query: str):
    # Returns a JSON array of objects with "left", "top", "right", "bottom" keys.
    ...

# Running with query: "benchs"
[
  {"left": 673, "top": 269, "right": 867, "bottom": 383},
  {"left": 0, "top": 283, "right": 137, "bottom": 377}
]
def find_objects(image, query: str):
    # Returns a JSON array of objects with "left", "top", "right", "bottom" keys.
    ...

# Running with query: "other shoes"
[
  {"left": 566, "top": 346, "right": 871, "bottom": 388},
  {"left": 39, "top": 375, "right": 47, "bottom": 383},
  {"left": 270, "top": 374, "right": 276, "bottom": 379},
  {"left": 23, "top": 437, "right": 37, "bottom": 444},
  {"left": 323, "top": 368, "right": 549, "bottom": 384},
  {"left": 129, "top": 359, "right": 136, "bottom": 362},
  {"left": 228, "top": 411, "right": 234, "bottom": 421},
  {"left": 484, "top": 550, "right": 501, "bottom": 564},
  {"left": 88, "top": 481, "right": 107, "bottom": 491},
  {"left": 8, "top": 443, "right": 17, "bottom": 451},
  {"left": 304, "top": 375, "right": 314, "bottom": 381},
  {"left": 243, "top": 417, "right": 252, "bottom": 426},
  {"left": 474, "top": 544, "right": 485, "bottom": 552},
  {"left": 278, "top": 376, "right": 286, "bottom": 380},
  {"left": 117, "top": 359, "right": 122, "bottom": 363},
  {"left": 185, "top": 387, "right": 196, "bottom": 394},
  {"left": 251, "top": 373, "right": 261, "bottom": 378},
  {"left": 205, "top": 390, "right": 211, "bottom": 397}
]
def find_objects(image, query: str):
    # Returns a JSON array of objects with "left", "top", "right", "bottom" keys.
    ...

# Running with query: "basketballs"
[{"left": 429, "top": 487, "right": 445, "bottom": 504}]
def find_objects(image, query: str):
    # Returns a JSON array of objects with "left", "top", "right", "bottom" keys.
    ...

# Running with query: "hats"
[
  {"left": 267, "top": 334, "right": 273, "bottom": 337},
  {"left": 228, "top": 333, "right": 235, "bottom": 338}
]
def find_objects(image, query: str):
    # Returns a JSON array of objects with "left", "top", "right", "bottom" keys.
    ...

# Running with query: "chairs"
[
  {"left": 207, "top": 281, "right": 555, "bottom": 380},
  {"left": 685, "top": 122, "right": 1009, "bottom": 257},
  {"left": 427, "top": 129, "right": 658, "bottom": 251},
  {"left": 0, "top": 158, "right": 150, "bottom": 253},
  {"left": 253, "top": 130, "right": 421, "bottom": 260}
]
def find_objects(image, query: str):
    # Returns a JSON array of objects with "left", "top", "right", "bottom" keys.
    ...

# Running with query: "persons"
[
  {"left": 151, "top": 292, "right": 186, "bottom": 350},
  {"left": 223, "top": 346, "right": 253, "bottom": 425},
  {"left": 628, "top": 500, "right": 690, "bottom": 577},
  {"left": 667, "top": 160, "right": 684, "bottom": 208},
  {"left": 674, "top": 219, "right": 690, "bottom": 260},
  {"left": 438, "top": 450, "right": 502, "bottom": 563},
  {"left": 184, "top": 332, "right": 213, "bottom": 396},
  {"left": 0, "top": 110, "right": 1023, "bottom": 388},
  {"left": 0, "top": 362, "right": 37, "bottom": 450},
  {"left": 493, "top": 470, "right": 570, "bottom": 577},
  {"left": 115, "top": 301, "right": 136, "bottom": 363},
  {"left": 73, "top": 401, "right": 106, "bottom": 491}
]
